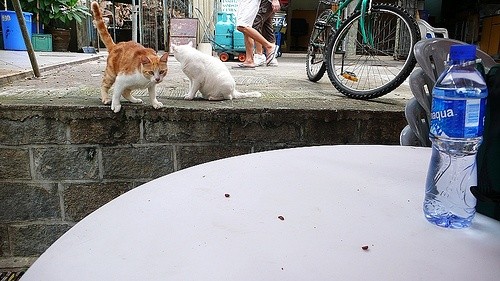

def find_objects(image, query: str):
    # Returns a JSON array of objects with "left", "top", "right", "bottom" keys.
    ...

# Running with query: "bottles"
[{"left": 423, "top": 42, "right": 488, "bottom": 230}]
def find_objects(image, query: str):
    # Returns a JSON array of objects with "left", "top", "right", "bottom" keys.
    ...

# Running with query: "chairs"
[
  {"left": 419, "top": 19, "right": 449, "bottom": 62},
  {"left": 400, "top": 38, "right": 496, "bottom": 148}
]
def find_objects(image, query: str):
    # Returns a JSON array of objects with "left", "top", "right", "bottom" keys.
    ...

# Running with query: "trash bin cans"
[{"left": 0, "top": 10, "right": 33, "bottom": 51}]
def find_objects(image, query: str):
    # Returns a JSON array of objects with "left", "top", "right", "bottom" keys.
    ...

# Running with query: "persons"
[
  {"left": 254, "top": 0, "right": 280, "bottom": 67},
  {"left": 232, "top": 0, "right": 279, "bottom": 68}
]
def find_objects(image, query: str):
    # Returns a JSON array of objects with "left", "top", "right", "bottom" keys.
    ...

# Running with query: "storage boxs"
[{"left": 31, "top": 34, "right": 52, "bottom": 51}]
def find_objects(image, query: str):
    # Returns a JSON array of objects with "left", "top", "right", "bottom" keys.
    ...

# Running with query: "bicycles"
[{"left": 304, "top": 0, "right": 420, "bottom": 101}]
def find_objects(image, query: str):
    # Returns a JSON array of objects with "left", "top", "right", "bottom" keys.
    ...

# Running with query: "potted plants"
[{"left": 20, "top": 0, "right": 93, "bottom": 52}]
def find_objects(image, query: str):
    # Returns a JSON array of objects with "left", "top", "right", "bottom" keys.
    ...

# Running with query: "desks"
[{"left": 18, "top": 145, "right": 500, "bottom": 281}]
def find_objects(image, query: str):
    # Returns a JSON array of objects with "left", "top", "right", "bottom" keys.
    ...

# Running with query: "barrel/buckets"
[{"left": 0, "top": 9, "right": 32, "bottom": 51}]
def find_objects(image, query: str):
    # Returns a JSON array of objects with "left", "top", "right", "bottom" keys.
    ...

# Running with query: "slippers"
[
  {"left": 233, "top": 63, "right": 255, "bottom": 70},
  {"left": 266, "top": 45, "right": 279, "bottom": 66}
]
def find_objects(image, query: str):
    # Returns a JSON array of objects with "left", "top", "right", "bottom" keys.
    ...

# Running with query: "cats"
[
  {"left": 91, "top": 1, "right": 168, "bottom": 113},
  {"left": 171, "top": 41, "right": 261, "bottom": 101}
]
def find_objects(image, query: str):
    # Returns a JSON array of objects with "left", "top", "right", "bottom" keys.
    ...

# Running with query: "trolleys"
[{"left": 193, "top": 5, "right": 257, "bottom": 61}]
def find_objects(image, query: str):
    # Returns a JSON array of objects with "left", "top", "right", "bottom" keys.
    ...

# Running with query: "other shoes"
[
  {"left": 269, "top": 58, "right": 278, "bottom": 66},
  {"left": 253, "top": 53, "right": 266, "bottom": 66}
]
